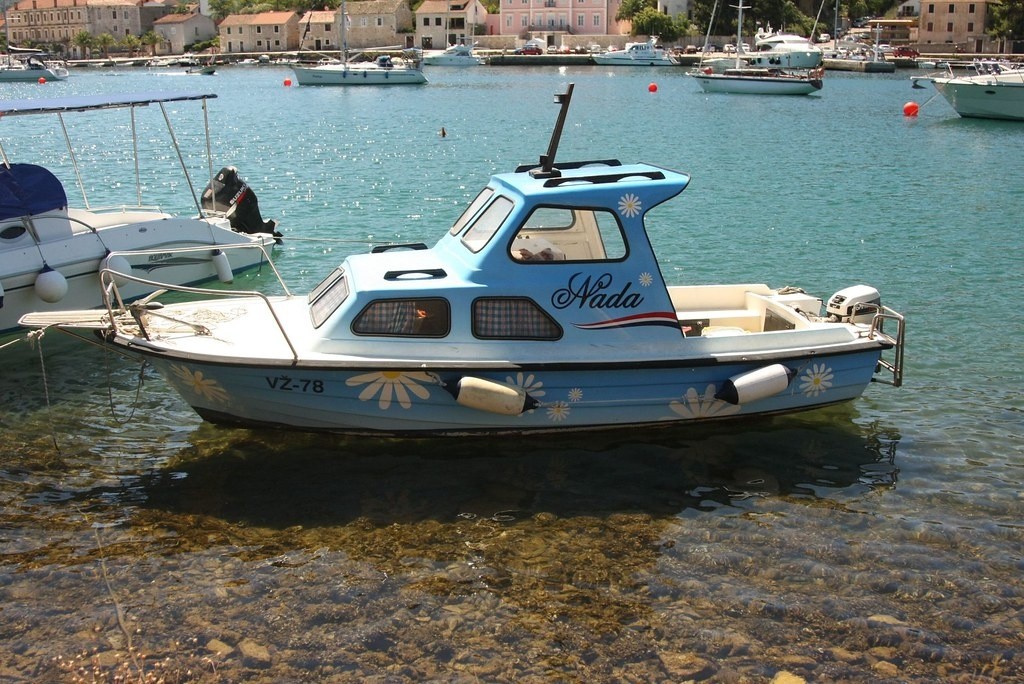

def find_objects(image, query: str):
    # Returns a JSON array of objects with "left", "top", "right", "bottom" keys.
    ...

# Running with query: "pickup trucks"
[{"left": 893, "top": 47, "right": 920, "bottom": 59}]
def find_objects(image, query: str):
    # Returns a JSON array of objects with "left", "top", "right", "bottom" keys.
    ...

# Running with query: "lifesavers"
[{"left": 819, "top": 68, "right": 824, "bottom": 79}]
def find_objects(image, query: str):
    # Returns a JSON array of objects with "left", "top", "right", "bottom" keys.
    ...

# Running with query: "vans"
[{"left": 521, "top": 46, "right": 542, "bottom": 55}]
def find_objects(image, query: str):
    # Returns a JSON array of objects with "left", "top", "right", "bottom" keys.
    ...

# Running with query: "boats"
[
  {"left": 145, "top": 59, "right": 198, "bottom": 67},
  {"left": 684, "top": 0, "right": 826, "bottom": 95},
  {"left": 185, "top": 66, "right": 215, "bottom": 76},
  {"left": 113, "top": 62, "right": 133, "bottom": 68},
  {"left": 417, "top": 41, "right": 480, "bottom": 66},
  {"left": 584, "top": 36, "right": 681, "bottom": 66},
  {"left": 910, "top": 58, "right": 1024, "bottom": 120},
  {"left": 18, "top": 83, "right": 905, "bottom": 459},
  {"left": 88, "top": 62, "right": 105, "bottom": 68},
  {"left": 0, "top": 94, "right": 283, "bottom": 332}
]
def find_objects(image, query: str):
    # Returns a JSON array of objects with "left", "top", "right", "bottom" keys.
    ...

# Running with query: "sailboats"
[
  {"left": 0, "top": 0, "right": 68, "bottom": 81},
  {"left": 288, "top": 0, "right": 429, "bottom": 85}
]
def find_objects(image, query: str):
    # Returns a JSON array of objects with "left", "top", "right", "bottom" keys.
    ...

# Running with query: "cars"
[
  {"left": 575, "top": 46, "right": 587, "bottom": 53},
  {"left": 607, "top": 45, "right": 618, "bottom": 52},
  {"left": 547, "top": 45, "right": 570, "bottom": 54},
  {"left": 655, "top": 43, "right": 751, "bottom": 54},
  {"left": 818, "top": 33, "right": 831, "bottom": 42},
  {"left": 820, "top": 16, "right": 893, "bottom": 61}
]
